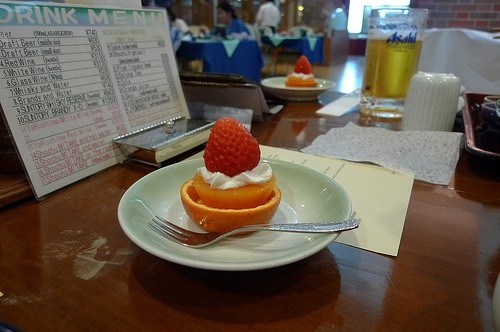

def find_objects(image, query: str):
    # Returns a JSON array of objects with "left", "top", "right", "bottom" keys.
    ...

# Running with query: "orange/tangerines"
[
  {"left": 180, "top": 171, "right": 282, "bottom": 236},
  {"left": 285, "top": 75, "right": 317, "bottom": 87}
]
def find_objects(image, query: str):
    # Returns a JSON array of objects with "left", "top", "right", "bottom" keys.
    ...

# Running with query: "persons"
[
  {"left": 255, "top": 0, "right": 282, "bottom": 33},
  {"left": 217, "top": 3, "right": 251, "bottom": 38},
  {"left": 166, "top": 6, "right": 188, "bottom": 32}
]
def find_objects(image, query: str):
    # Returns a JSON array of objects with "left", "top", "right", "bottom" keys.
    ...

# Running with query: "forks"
[{"left": 146, "top": 214, "right": 361, "bottom": 250}]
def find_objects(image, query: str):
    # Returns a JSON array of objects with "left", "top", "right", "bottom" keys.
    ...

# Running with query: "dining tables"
[{"left": 0, "top": 90, "right": 500, "bottom": 332}]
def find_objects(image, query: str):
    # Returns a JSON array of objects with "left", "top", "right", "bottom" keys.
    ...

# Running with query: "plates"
[
  {"left": 260, "top": 77, "right": 336, "bottom": 101},
  {"left": 115, "top": 156, "right": 352, "bottom": 271}
]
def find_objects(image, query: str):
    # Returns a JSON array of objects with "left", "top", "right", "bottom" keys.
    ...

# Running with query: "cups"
[
  {"left": 400, "top": 70, "right": 461, "bottom": 135},
  {"left": 482, "top": 95, "right": 500, "bottom": 123},
  {"left": 359, "top": 6, "right": 428, "bottom": 118}
]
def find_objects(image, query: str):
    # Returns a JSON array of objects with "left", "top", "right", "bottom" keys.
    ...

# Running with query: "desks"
[
  {"left": 213, "top": 24, "right": 254, "bottom": 29},
  {"left": 273, "top": 36, "right": 324, "bottom": 74},
  {"left": 176, "top": 36, "right": 264, "bottom": 83}
]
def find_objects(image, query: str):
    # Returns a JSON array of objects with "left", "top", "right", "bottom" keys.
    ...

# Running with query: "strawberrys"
[
  {"left": 202, "top": 117, "right": 261, "bottom": 176},
  {"left": 294, "top": 55, "right": 312, "bottom": 74}
]
